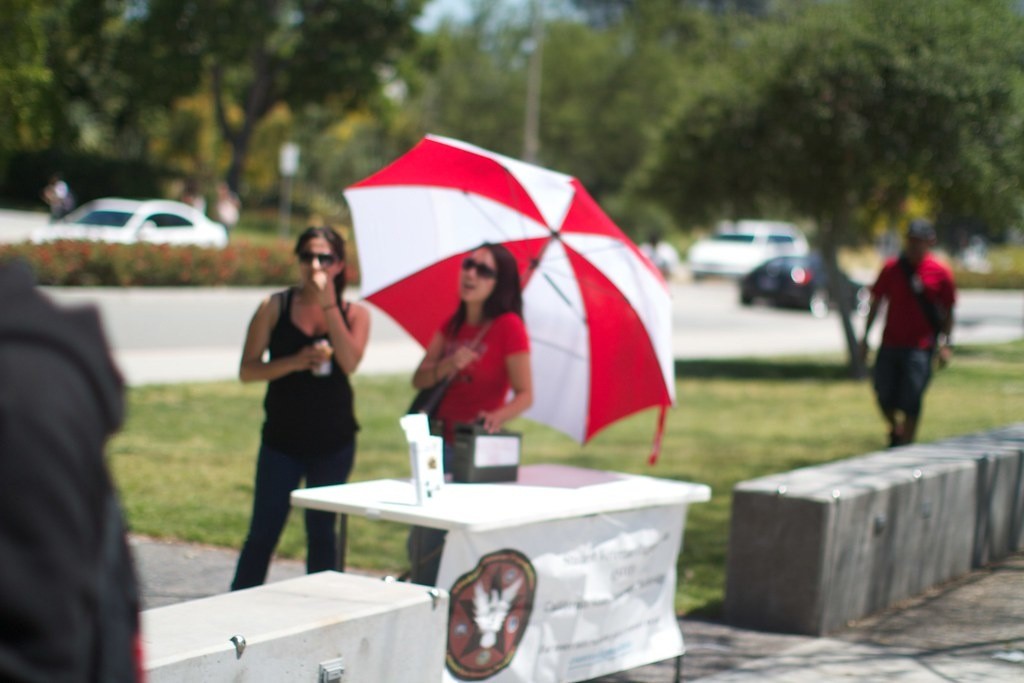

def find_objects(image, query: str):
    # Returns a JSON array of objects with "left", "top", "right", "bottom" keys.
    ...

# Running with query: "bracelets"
[
  {"left": 323, "top": 303, "right": 339, "bottom": 311},
  {"left": 432, "top": 362, "right": 440, "bottom": 386}
]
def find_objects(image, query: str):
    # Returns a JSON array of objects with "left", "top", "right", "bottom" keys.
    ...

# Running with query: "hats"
[{"left": 907, "top": 221, "right": 934, "bottom": 240}]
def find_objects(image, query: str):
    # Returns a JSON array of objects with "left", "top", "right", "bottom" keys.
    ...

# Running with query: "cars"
[
  {"left": 687, "top": 219, "right": 803, "bottom": 285},
  {"left": 31, "top": 198, "right": 229, "bottom": 252},
  {"left": 742, "top": 256, "right": 872, "bottom": 317}
]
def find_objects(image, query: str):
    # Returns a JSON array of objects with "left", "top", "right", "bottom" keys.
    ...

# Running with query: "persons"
[
  {"left": 229, "top": 225, "right": 371, "bottom": 592},
  {"left": 44, "top": 165, "right": 241, "bottom": 236},
  {"left": 407, "top": 243, "right": 534, "bottom": 585},
  {"left": 858, "top": 218, "right": 956, "bottom": 447},
  {"left": 0, "top": 245, "right": 146, "bottom": 683}
]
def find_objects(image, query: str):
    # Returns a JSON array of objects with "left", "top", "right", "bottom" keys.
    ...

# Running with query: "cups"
[{"left": 311, "top": 340, "right": 332, "bottom": 375}]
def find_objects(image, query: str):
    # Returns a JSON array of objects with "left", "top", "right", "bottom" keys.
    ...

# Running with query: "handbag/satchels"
[
  {"left": 922, "top": 295, "right": 949, "bottom": 331},
  {"left": 408, "top": 380, "right": 447, "bottom": 415}
]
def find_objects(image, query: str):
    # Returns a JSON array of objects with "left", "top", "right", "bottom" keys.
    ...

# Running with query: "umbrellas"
[{"left": 342, "top": 135, "right": 677, "bottom": 465}]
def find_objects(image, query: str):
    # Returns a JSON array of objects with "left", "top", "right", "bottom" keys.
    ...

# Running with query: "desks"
[{"left": 289, "top": 463, "right": 711, "bottom": 683}]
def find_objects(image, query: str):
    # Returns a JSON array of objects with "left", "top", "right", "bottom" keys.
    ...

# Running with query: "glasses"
[
  {"left": 300, "top": 251, "right": 338, "bottom": 266},
  {"left": 462, "top": 256, "right": 497, "bottom": 280}
]
